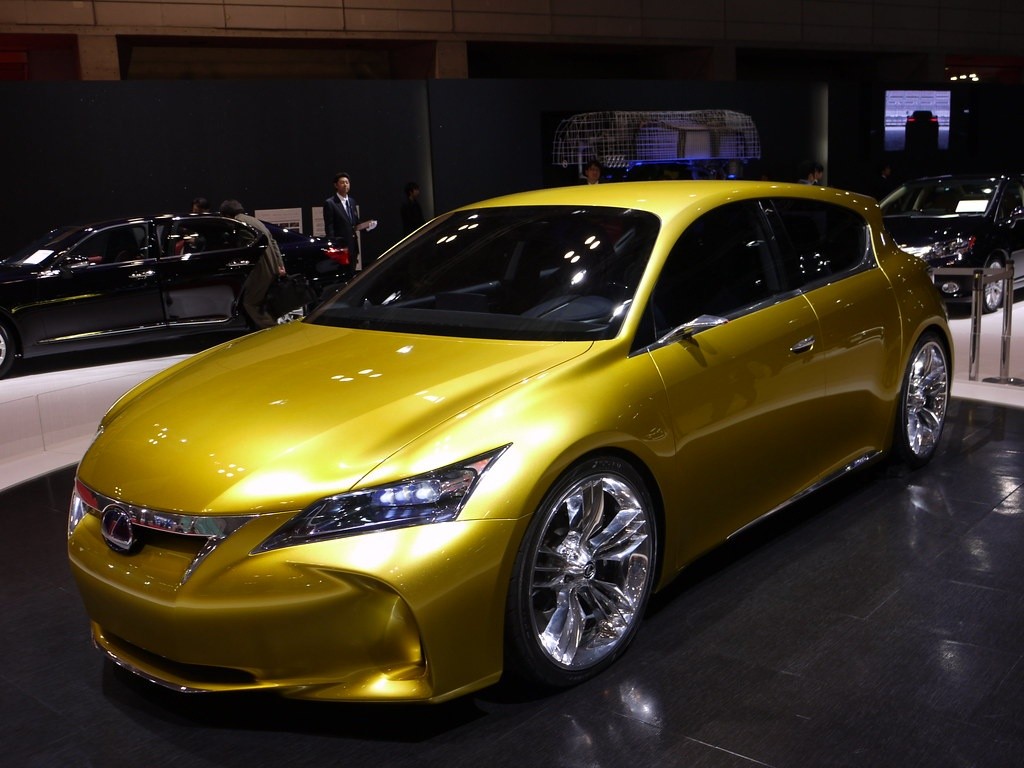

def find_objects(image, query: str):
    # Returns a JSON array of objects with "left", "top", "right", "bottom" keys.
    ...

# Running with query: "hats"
[{"left": 220, "top": 199, "right": 243, "bottom": 211}]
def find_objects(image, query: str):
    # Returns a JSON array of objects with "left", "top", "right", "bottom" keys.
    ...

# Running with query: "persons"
[
  {"left": 323, "top": 172, "right": 361, "bottom": 279},
  {"left": 183, "top": 197, "right": 285, "bottom": 330},
  {"left": 583, "top": 160, "right": 602, "bottom": 186},
  {"left": 401, "top": 181, "right": 427, "bottom": 236},
  {"left": 878, "top": 166, "right": 891, "bottom": 201},
  {"left": 797, "top": 159, "right": 824, "bottom": 185}
]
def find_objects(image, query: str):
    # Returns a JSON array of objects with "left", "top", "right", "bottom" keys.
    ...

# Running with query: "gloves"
[{"left": 366, "top": 221, "right": 377, "bottom": 231}]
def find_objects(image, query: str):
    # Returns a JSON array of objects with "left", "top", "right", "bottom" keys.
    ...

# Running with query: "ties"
[{"left": 346, "top": 200, "right": 351, "bottom": 218}]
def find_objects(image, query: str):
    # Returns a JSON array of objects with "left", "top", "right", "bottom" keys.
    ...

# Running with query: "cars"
[
  {"left": 67, "top": 179, "right": 956, "bottom": 705},
  {"left": 0, "top": 211, "right": 351, "bottom": 379},
  {"left": 879, "top": 170, "right": 1024, "bottom": 313}
]
